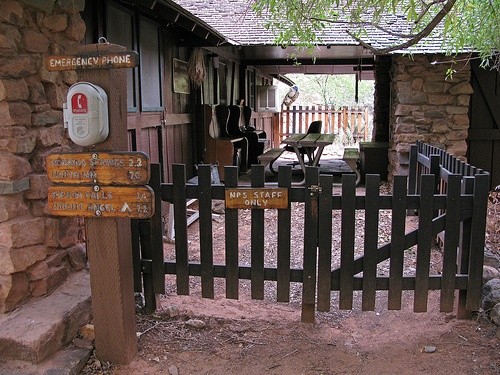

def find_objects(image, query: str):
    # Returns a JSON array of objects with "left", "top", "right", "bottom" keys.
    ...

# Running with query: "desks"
[
  {"left": 281, "top": 133, "right": 337, "bottom": 186},
  {"left": 243, "top": 130, "right": 267, "bottom": 166},
  {"left": 201, "top": 137, "right": 249, "bottom": 182}
]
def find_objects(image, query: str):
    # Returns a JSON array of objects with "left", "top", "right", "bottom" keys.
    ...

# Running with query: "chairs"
[{"left": 282, "top": 120, "right": 322, "bottom": 167}]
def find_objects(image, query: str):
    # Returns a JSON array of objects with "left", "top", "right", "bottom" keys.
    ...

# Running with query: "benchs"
[
  {"left": 342, "top": 147, "right": 362, "bottom": 187},
  {"left": 258, "top": 147, "right": 285, "bottom": 182}
]
